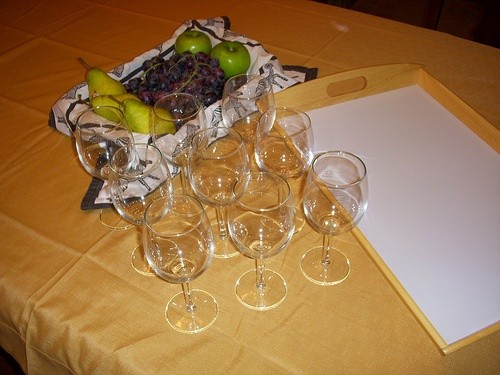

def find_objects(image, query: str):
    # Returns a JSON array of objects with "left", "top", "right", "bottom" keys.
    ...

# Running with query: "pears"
[{"left": 79, "top": 58, "right": 177, "bottom": 135}]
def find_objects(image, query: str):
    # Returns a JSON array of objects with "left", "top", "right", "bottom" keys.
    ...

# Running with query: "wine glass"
[
  {"left": 187, "top": 126, "right": 248, "bottom": 259},
  {"left": 254, "top": 107, "right": 314, "bottom": 233},
  {"left": 222, "top": 73, "right": 277, "bottom": 192},
  {"left": 74, "top": 105, "right": 146, "bottom": 233},
  {"left": 226, "top": 171, "right": 296, "bottom": 312},
  {"left": 299, "top": 150, "right": 369, "bottom": 286},
  {"left": 141, "top": 195, "right": 219, "bottom": 334},
  {"left": 109, "top": 144, "right": 180, "bottom": 277},
  {"left": 151, "top": 93, "right": 208, "bottom": 216}
]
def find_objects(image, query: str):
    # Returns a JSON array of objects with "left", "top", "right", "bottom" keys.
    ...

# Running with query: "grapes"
[{"left": 128, "top": 50, "right": 226, "bottom": 129}]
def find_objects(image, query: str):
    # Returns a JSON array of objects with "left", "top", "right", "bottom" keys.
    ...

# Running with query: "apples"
[
  {"left": 175, "top": 30, "right": 212, "bottom": 56},
  {"left": 211, "top": 41, "right": 251, "bottom": 78}
]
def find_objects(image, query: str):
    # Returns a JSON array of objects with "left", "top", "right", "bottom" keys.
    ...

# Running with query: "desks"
[{"left": 0, "top": 0, "right": 500, "bottom": 375}]
